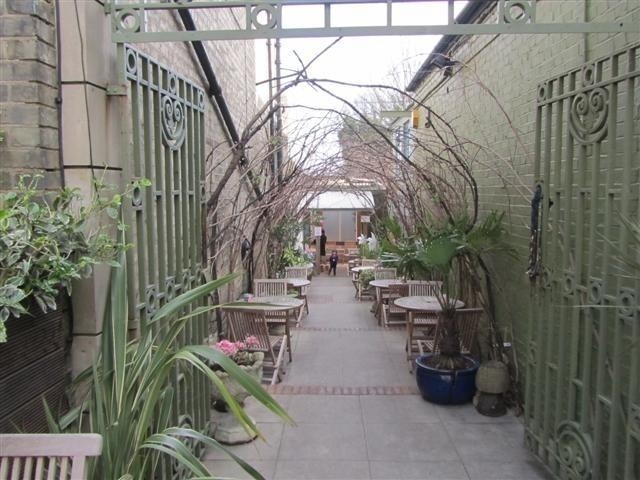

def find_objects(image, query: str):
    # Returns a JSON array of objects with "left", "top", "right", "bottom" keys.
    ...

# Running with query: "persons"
[
  {"left": 328, "top": 249, "right": 338, "bottom": 277},
  {"left": 320, "top": 229, "right": 326, "bottom": 256}
]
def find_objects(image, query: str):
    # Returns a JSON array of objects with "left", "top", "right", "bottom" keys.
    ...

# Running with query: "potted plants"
[{"left": 377, "top": 206, "right": 522, "bottom": 404}]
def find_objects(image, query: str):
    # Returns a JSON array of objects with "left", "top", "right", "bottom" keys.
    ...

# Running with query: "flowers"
[{"left": 209, "top": 334, "right": 261, "bottom": 371}]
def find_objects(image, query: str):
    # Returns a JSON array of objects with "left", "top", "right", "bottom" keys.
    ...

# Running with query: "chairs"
[
  {"left": 222, "top": 267, "right": 311, "bottom": 385},
  {"left": 348, "top": 259, "right": 484, "bottom": 374},
  {"left": 0, "top": 433, "right": 103, "bottom": 480}
]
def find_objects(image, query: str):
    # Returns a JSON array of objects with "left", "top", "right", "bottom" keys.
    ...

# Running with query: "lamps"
[{"left": 430, "top": 55, "right": 452, "bottom": 69}]
